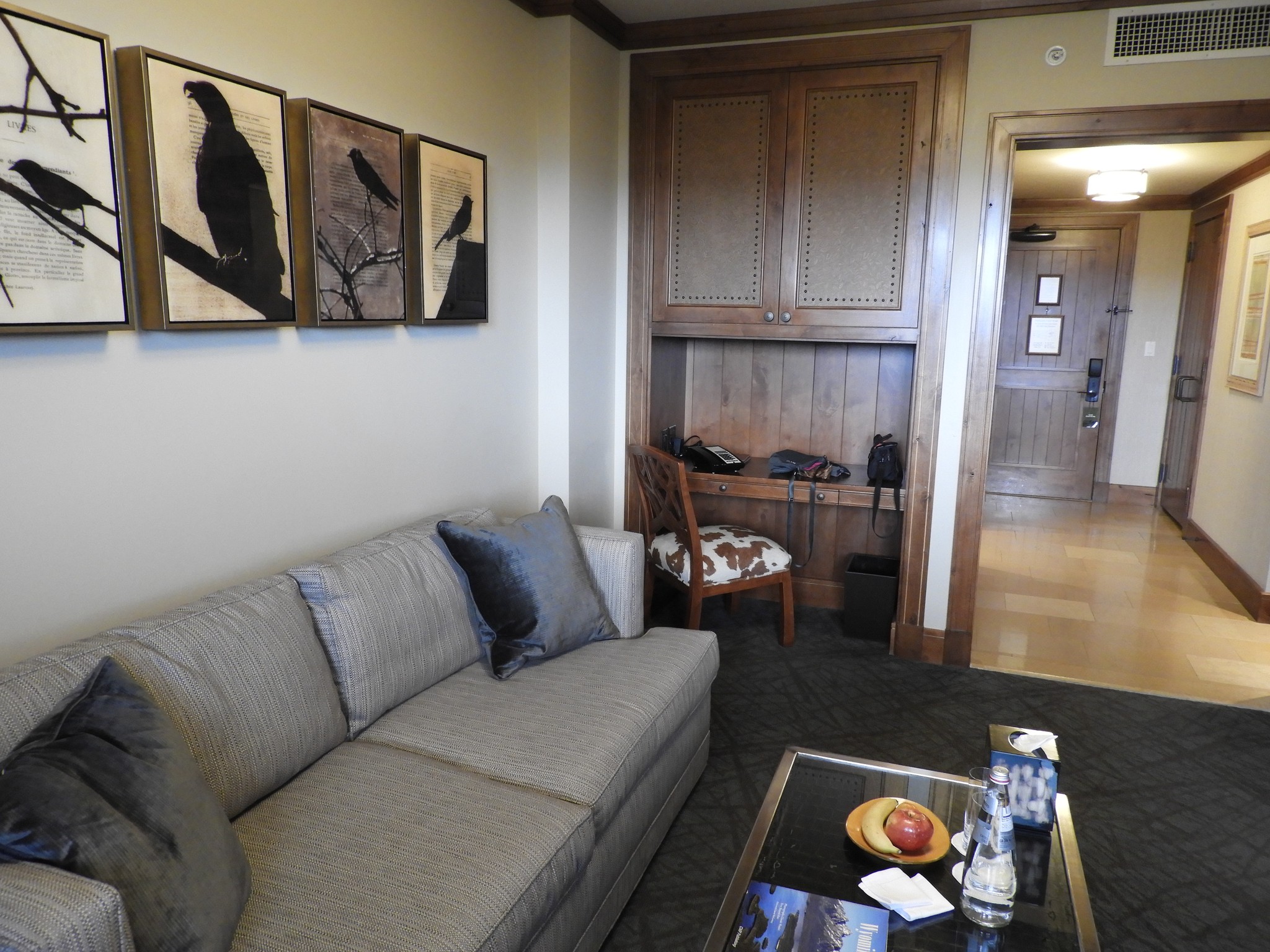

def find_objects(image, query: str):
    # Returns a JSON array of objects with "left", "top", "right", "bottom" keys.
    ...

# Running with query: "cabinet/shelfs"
[{"left": 653, "top": 53, "right": 941, "bottom": 328}]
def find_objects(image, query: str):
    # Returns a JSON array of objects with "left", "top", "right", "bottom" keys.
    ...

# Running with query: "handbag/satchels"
[
  {"left": 867, "top": 434, "right": 903, "bottom": 538},
  {"left": 768, "top": 449, "right": 829, "bottom": 568}
]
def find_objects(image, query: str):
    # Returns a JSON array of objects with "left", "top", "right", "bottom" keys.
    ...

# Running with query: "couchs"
[{"left": 0, "top": 512, "right": 720, "bottom": 952}]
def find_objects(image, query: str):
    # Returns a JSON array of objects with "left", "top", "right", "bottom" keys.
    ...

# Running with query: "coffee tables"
[{"left": 703, "top": 744, "right": 1101, "bottom": 952}]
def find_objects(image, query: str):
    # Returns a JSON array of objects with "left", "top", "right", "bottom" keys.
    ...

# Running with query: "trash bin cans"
[{"left": 842, "top": 552, "right": 899, "bottom": 641}]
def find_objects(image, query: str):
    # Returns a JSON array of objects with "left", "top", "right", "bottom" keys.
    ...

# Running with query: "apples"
[
  {"left": 897, "top": 802, "right": 919, "bottom": 811},
  {"left": 886, "top": 809, "right": 934, "bottom": 850}
]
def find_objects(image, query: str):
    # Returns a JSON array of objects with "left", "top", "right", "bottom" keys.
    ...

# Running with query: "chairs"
[{"left": 630, "top": 442, "right": 795, "bottom": 647}]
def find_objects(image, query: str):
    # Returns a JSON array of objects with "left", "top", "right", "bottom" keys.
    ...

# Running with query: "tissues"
[{"left": 987, "top": 723, "right": 1062, "bottom": 833}]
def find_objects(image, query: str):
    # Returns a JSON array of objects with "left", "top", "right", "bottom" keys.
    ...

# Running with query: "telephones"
[{"left": 686, "top": 444, "right": 745, "bottom": 472}]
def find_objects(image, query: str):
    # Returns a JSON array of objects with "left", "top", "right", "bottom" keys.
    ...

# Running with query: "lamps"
[{"left": 1086, "top": 168, "right": 1148, "bottom": 202}]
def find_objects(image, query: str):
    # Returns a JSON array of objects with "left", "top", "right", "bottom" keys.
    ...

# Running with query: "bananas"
[{"left": 861, "top": 799, "right": 902, "bottom": 854}]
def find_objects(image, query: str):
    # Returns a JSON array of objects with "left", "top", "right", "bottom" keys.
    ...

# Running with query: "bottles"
[{"left": 960, "top": 766, "right": 1018, "bottom": 928}]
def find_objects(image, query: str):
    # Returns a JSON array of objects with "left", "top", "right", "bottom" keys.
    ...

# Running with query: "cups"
[{"left": 962, "top": 768, "right": 991, "bottom": 852}]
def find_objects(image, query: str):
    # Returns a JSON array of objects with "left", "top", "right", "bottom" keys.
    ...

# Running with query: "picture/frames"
[
  {"left": 0, "top": 0, "right": 136, "bottom": 334},
  {"left": 1225, "top": 219, "right": 1270, "bottom": 397},
  {"left": 116, "top": 46, "right": 299, "bottom": 329},
  {"left": 404, "top": 132, "right": 490, "bottom": 324},
  {"left": 288, "top": 96, "right": 409, "bottom": 326}
]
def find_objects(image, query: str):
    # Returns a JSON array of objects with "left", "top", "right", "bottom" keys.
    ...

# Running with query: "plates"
[{"left": 846, "top": 797, "right": 951, "bottom": 864}]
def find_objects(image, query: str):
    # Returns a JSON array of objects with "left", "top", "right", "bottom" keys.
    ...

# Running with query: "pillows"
[
  {"left": 437, "top": 496, "right": 621, "bottom": 681},
  {"left": 0, "top": 657, "right": 254, "bottom": 952}
]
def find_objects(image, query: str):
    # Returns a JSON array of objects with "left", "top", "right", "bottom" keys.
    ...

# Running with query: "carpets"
[{"left": 601, "top": 592, "right": 1270, "bottom": 952}]
